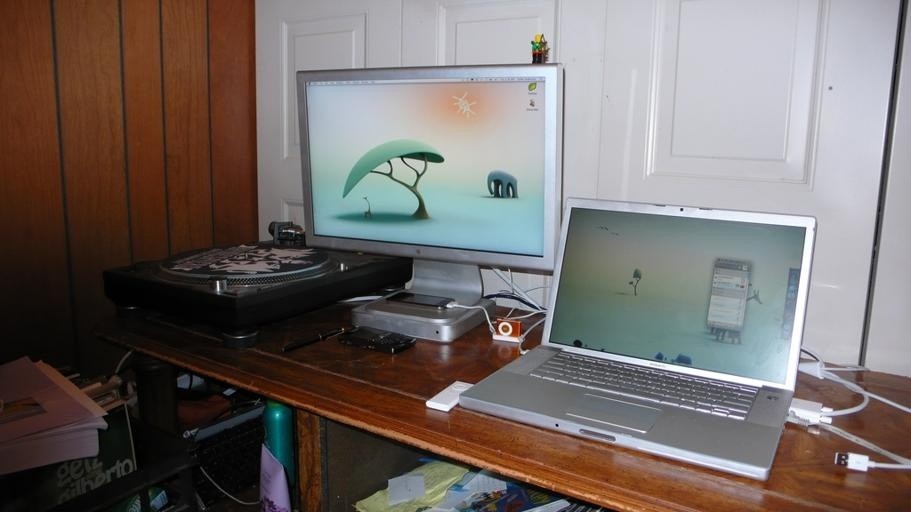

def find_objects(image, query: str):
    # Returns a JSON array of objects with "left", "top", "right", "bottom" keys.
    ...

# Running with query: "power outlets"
[{"left": 492, "top": 319, "right": 525, "bottom": 343}]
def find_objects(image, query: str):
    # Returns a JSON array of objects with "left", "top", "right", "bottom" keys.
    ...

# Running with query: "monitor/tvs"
[{"left": 296, "top": 65, "right": 565, "bottom": 344}]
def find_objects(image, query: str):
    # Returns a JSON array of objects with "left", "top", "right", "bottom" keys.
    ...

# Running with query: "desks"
[{"left": 123, "top": 305, "right": 911, "bottom": 511}]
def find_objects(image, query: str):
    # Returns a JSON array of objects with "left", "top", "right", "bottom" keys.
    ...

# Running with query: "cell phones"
[
  {"left": 340, "top": 324, "right": 419, "bottom": 354},
  {"left": 386, "top": 291, "right": 454, "bottom": 307}
]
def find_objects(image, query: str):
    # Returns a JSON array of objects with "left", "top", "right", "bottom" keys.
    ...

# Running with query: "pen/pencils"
[{"left": 282, "top": 326, "right": 343, "bottom": 352}]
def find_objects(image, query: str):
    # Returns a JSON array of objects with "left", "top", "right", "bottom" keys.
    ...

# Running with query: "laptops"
[{"left": 458, "top": 196, "right": 819, "bottom": 484}]
